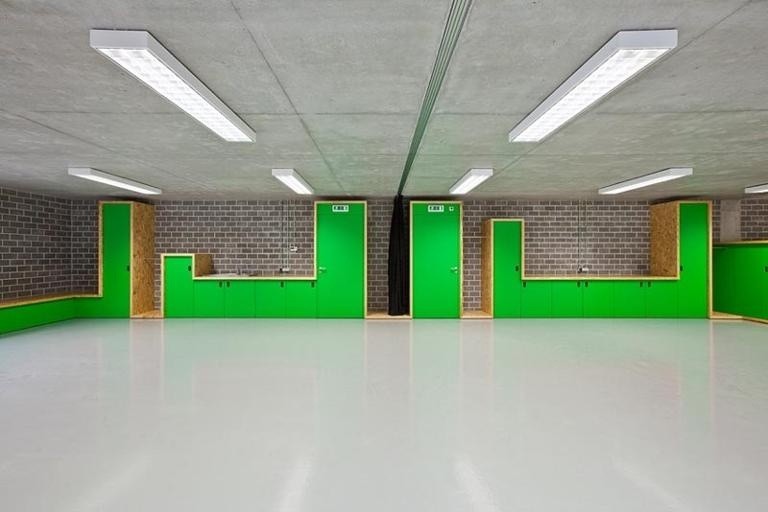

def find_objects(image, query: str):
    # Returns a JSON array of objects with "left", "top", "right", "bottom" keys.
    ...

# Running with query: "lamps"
[
  {"left": 89, "top": 30, "right": 257, "bottom": 143},
  {"left": 745, "top": 183, "right": 768, "bottom": 196},
  {"left": 70, "top": 167, "right": 162, "bottom": 196},
  {"left": 272, "top": 168, "right": 314, "bottom": 195},
  {"left": 598, "top": 168, "right": 691, "bottom": 194},
  {"left": 508, "top": 30, "right": 678, "bottom": 142},
  {"left": 448, "top": 168, "right": 494, "bottom": 194}
]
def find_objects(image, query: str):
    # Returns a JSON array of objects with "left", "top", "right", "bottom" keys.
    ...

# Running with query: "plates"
[
  {"left": 317, "top": 203, "right": 363, "bottom": 319},
  {"left": 522, "top": 281, "right": 551, "bottom": 318},
  {"left": 413, "top": 204, "right": 461, "bottom": 319},
  {"left": 495, "top": 222, "right": 522, "bottom": 318},
  {"left": 651, "top": 204, "right": 708, "bottom": 318},
  {"left": 99, "top": 202, "right": 156, "bottom": 317},
  {"left": 256, "top": 280, "right": 317, "bottom": 317},
  {"left": 193, "top": 280, "right": 256, "bottom": 317},
  {"left": 550, "top": 280, "right": 678, "bottom": 317},
  {"left": 163, "top": 256, "right": 214, "bottom": 317},
  {"left": 712, "top": 245, "right": 768, "bottom": 322}
]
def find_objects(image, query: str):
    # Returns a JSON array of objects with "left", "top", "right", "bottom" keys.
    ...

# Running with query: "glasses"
[{"left": 745, "top": 183, "right": 768, "bottom": 196}]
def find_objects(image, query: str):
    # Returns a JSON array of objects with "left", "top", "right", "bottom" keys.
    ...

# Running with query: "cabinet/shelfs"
[
  {"left": 163, "top": 256, "right": 214, "bottom": 317},
  {"left": 495, "top": 222, "right": 522, "bottom": 318},
  {"left": 256, "top": 280, "right": 317, "bottom": 317},
  {"left": 550, "top": 280, "right": 678, "bottom": 317},
  {"left": 317, "top": 203, "right": 363, "bottom": 319},
  {"left": 522, "top": 281, "right": 551, "bottom": 318},
  {"left": 413, "top": 204, "right": 461, "bottom": 319},
  {"left": 193, "top": 280, "right": 256, "bottom": 317},
  {"left": 712, "top": 245, "right": 768, "bottom": 322},
  {"left": 651, "top": 204, "right": 708, "bottom": 318},
  {"left": 99, "top": 202, "right": 156, "bottom": 317}
]
